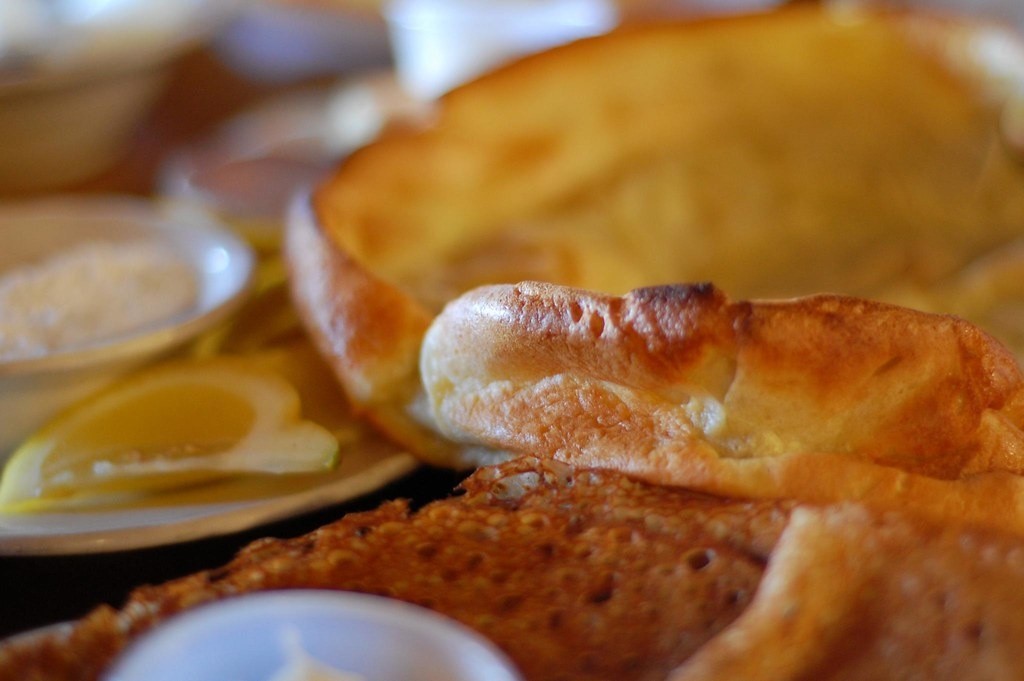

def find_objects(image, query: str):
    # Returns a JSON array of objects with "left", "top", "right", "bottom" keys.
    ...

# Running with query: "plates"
[{"left": 0, "top": 438, "right": 417, "bottom": 557}]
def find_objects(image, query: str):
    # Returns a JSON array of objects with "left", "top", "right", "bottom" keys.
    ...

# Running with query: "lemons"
[{"left": 4, "top": 359, "right": 308, "bottom": 499}]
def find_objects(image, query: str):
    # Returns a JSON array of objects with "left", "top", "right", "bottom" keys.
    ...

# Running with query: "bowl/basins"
[
  {"left": 0, "top": 196, "right": 254, "bottom": 446},
  {"left": 101, "top": 590, "right": 521, "bottom": 681}
]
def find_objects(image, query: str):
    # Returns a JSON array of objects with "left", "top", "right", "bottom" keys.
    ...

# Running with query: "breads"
[{"left": 0, "top": 0, "right": 1024, "bottom": 681}]
